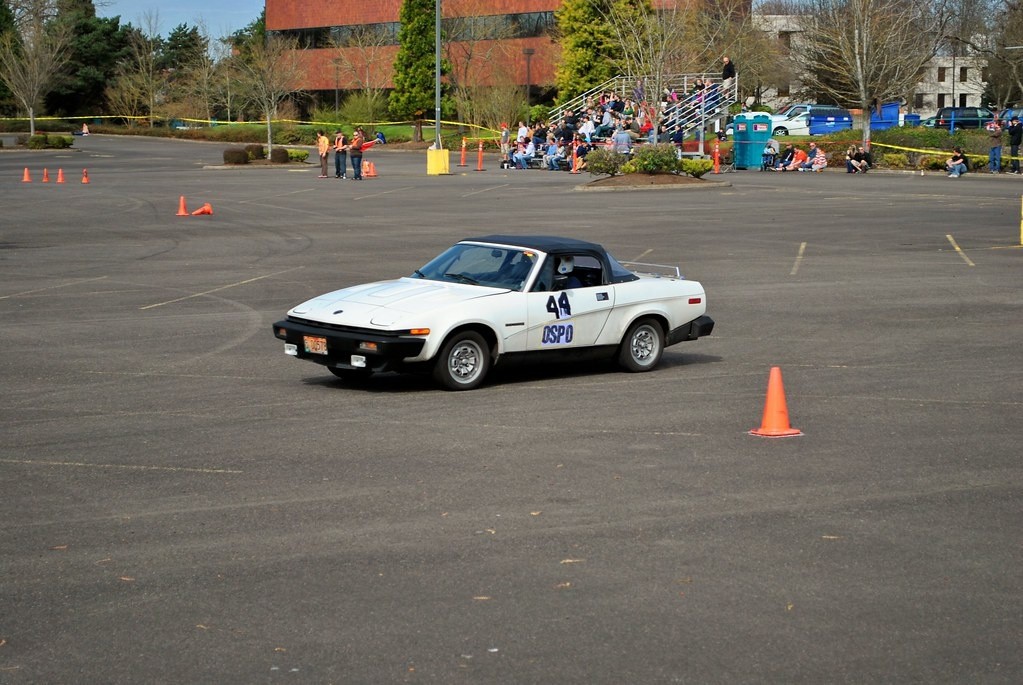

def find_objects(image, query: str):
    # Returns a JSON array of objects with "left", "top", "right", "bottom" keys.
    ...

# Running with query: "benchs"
[
  {"left": 509, "top": 93, "right": 724, "bottom": 171},
  {"left": 574, "top": 268, "right": 603, "bottom": 285}
]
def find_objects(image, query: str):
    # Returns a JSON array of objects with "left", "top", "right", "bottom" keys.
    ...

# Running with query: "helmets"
[{"left": 557, "top": 255, "right": 575, "bottom": 274}]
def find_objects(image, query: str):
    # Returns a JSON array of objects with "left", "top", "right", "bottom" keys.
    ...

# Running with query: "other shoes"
[
  {"left": 1006, "top": 170, "right": 1020, "bottom": 175},
  {"left": 318, "top": 174, "right": 363, "bottom": 180},
  {"left": 948, "top": 174, "right": 958, "bottom": 178},
  {"left": 782, "top": 167, "right": 786, "bottom": 171},
  {"left": 771, "top": 168, "right": 777, "bottom": 172}
]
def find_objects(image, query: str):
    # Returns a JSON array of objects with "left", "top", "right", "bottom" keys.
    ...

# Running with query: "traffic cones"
[
  {"left": 749, "top": 367, "right": 801, "bottom": 436},
  {"left": 191, "top": 203, "right": 213, "bottom": 215},
  {"left": 175, "top": 196, "right": 189, "bottom": 216},
  {"left": 56, "top": 169, "right": 65, "bottom": 183},
  {"left": 21, "top": 168, "right": 32, "bottom": 181},
  {"left": 81, "top": 168, "right": 90, "bottom": 184},
  {"left": 360, "top": 160, "right": 378, "bottom": 177},
  {"left": 42, "top": 169, "right": 49, "bottom": 181}
]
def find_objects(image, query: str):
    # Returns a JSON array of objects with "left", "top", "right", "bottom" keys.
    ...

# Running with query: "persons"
[
  {"left": 500, "top": 57, "right": 747, "bottom": 174},
  {"left": 316, "top": 128, "right": 331, "bottom": 178},
  {"left": 1008, "top": 116, "right": 1023, "bottom": 174},
  {"left": 760, "top": 140, "right": 873, "bottom": 173},
  {"left": 350, "top": 131, "right": 363, "bottom": 180},
  {"left": 946, "top": 146, "right": 969, "bottom": 178},
  {"left": 989, "top": 120, "right": 1002, "bottom": 174},
  {"left": 332, "top": 128, "right": 349, "bottom": 178},
  {"left": 356, "top": 126, "right": 386, "bottom": 145},
  {"left": 535, "top": 255, "right": 580, "bottom": 294}
]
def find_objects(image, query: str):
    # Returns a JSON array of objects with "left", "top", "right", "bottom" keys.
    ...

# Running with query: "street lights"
[
  {"left": 522, "top": 48, "right": 535, "bottom": 133},
  {"left": 333, "top": 58, "right": 342, "bottom": 122}
]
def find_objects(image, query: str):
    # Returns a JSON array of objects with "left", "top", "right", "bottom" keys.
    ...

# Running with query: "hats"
[
  {"left": 501, "top": 123, "right": 506, "bottom": 127},
  {"left": 1010, "top": 116, "right": 1018, "bottom": 122}
]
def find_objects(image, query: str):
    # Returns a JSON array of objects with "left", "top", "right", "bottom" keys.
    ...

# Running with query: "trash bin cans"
[
  {"left": 733, "top": 115, "right": 772, "bottom": 168},
  {"left": 425, "top": 148, "right": 450, "bottom": 175},
  {"left": 904, "top": 114, "right": 921, "bottom": 126}
]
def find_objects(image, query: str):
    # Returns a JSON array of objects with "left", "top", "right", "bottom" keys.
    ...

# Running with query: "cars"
[
  {"left": 772, "top": 112, "right": 824, "bottom": 136},
  {"left": 272, "top": 234, "right": 715, "bottom": 391},
  {"left": 771, "top": 104, "right": 840, "bottom": 123},
  {"left": 920, "top": 117, "right": 936, "bottom": 127},
  {"left": 997, "top": 108, "right": 1023, "bottom": 129}
]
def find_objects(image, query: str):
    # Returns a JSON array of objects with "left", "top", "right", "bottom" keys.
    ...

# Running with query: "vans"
[{"left": 934, "top": 107, "right": 994, "bottom": 130}]
